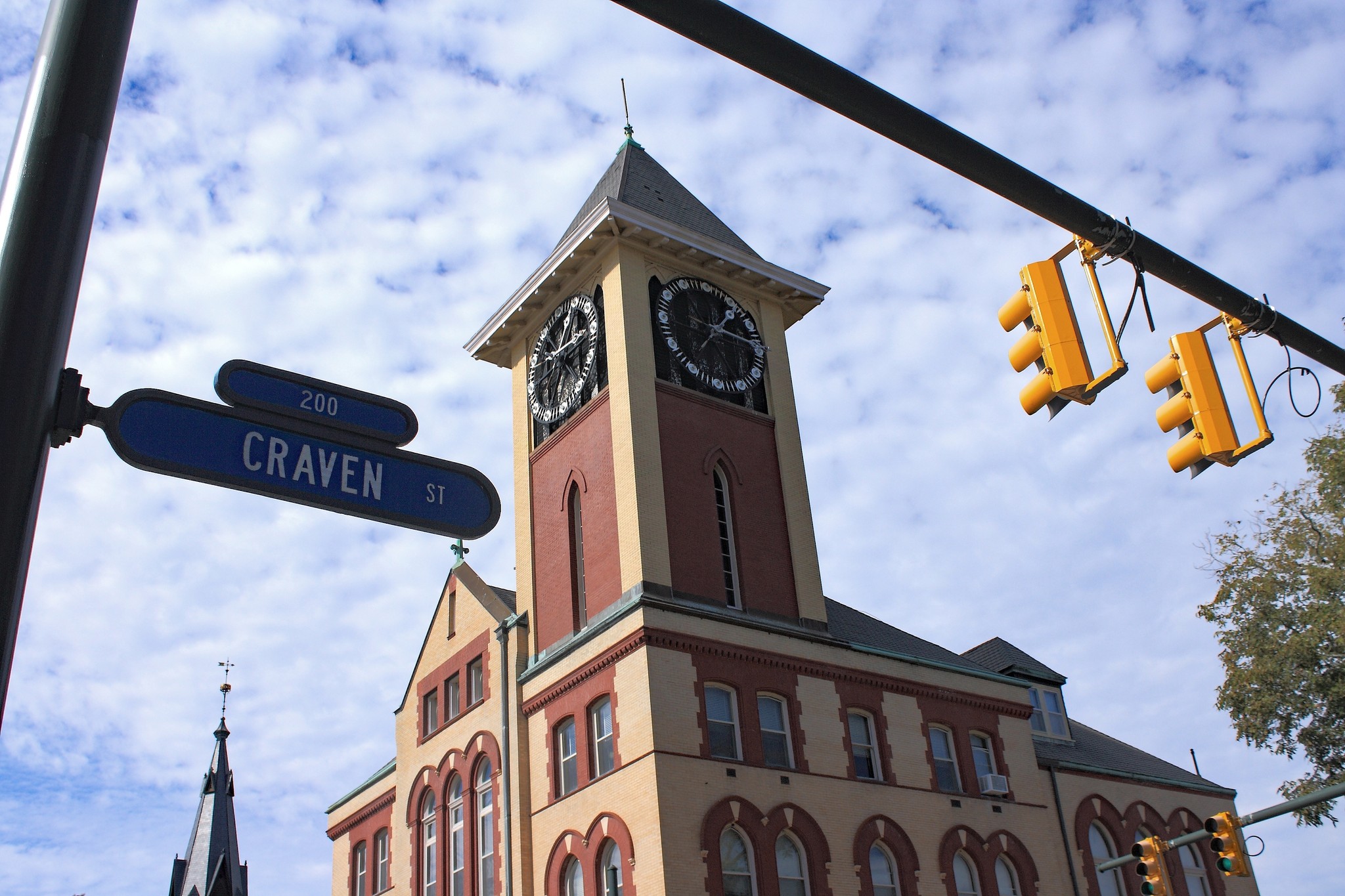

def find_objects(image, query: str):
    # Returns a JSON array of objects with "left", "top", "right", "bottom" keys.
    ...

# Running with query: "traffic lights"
[
  {"left": 1145, "top": 313, "right": 1272, "bottom": 481},
  {"left": 1131, "top": 836, "right": 1175, "bottom": 896},
  {"left": 998, "top": 260, "right": 1096, "bottom": 420},
  {"left": 1205, "top": 812, "right": 1247, "bottom": 876}
]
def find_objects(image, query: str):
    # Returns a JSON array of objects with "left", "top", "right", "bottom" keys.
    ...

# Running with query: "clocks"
[
  {"left": 654, "top": 277, "right": 773, "bottom": 395},
  {"left": 526, "top": 293, "right": 599, "bottom": 421}
]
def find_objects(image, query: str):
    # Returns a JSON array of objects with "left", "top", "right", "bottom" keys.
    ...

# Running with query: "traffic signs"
[{"left": 106, "top": 356, "right": 503, "bottom": 539}]
